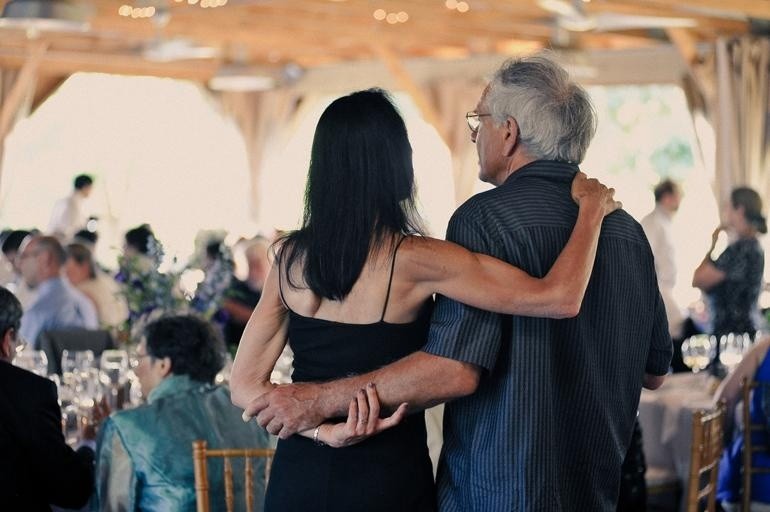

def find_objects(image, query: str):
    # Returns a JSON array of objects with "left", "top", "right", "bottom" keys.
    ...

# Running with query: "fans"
[{"left": 527, "top": 0, "right": 709, "bottom": 42}]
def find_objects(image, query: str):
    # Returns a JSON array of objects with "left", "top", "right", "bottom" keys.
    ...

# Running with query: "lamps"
[{"left": 0, "top": 1, "right": 93, "bottom": 36}]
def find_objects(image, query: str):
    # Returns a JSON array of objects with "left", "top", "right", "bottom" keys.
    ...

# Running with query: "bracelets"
[{"left": 313, "top": 425, "right": 327, "bottom": 446}]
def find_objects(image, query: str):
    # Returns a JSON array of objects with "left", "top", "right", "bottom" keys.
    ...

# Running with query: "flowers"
[{"left": 113, "top": 232, "right": 248, "bottom": 357}]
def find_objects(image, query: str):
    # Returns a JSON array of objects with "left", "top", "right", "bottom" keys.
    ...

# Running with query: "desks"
[{"left": 634, "top": 369, "right": 735, "bottom": 484}]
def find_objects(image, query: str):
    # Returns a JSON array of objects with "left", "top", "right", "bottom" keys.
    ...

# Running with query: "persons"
[
  {"left": 242, "top": 55, "right": 672, "bottom": 511},
  {"left": 637, "top": 176, "right": 769, "bottom": 505},
  {"left": 229, "top": 85, "right": 623, "bottom": 511},
  {"left": 1, "top": 171, "right": 297, "bottom": 512}
]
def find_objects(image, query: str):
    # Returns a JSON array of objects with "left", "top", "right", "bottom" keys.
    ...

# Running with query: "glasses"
[
  {"left": 465, "top": 110, "right": 492, "bottom": 133},
  {"left": 127, "top": 349, "right": 156, "bottom": 370},
  {"left": 13, "top": 329, "right": 28, "bottom": 353}
]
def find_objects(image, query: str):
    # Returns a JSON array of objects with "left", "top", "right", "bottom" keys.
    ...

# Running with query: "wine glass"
[{"left": 680, "top": 331, "right": 750, "bottom": 386}]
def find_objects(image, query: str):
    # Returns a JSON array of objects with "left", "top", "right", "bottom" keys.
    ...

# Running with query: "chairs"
[
  {"left": 189, "top": 438, "right": 279, "bottom": 512},
  {"left": 687, "top": 394, "right": 731, "bottom": 512},
  {"left": 736, "top": 373, "right": 770, "bottom": 511},
  {"left": 35, "top": 324, "right": 115, "bottom": 376}
]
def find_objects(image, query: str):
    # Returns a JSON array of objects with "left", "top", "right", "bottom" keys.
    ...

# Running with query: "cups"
[{"left": 14, "top": 348, "right": 142, "bottom": 443}]
